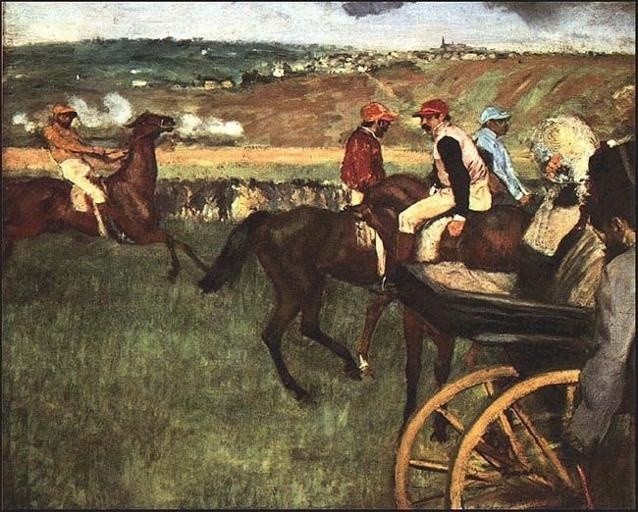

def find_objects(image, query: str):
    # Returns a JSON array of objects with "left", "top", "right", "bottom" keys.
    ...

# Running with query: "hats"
[
  {"left": 51, "top": 102, "right": 77, "bottom": 116},
  {"left": 412, "top": 98, "right": 450, "bottom": 119},
  {"left": 480, "top": 106, "right": 513, "bottom": 125},
  {"left": 359, "top": 101, "right": 401, "bottom": 124}
]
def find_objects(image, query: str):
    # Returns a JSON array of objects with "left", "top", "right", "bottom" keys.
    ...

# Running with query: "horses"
[
  {"left": 194, "top": 200, "right": 400, "bottom": 411},
  {"left": 343, "top": 172, "right": 538, "bottom": 449},
  {"left": 0, "top": 107, "right": 209, "bottom": 284}
]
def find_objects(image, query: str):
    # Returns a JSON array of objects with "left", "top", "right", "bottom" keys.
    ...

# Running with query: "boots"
[
  {"left": 365, "top": 232, "right": 418, "bottom": 295},
  {"left": 92, "top": 199, "right": 138, "bottom": 246}
]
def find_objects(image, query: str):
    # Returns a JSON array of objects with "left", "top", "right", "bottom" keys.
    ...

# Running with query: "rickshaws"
[{"left": 383, "top": 258, "right": 636, "bottom": 511}]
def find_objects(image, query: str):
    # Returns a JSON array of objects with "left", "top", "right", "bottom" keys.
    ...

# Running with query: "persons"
[
  {"left": 560, "top": 137, "right": 638, "bottom": 511},
  {"left": 42, "top": 103, "right": 136, "bottom": 245},
  {"left": 471, "top": 105, "right": 535, "bottom": 208},
  {"left": 393, "top": 98, "right": 490, "bottom": 262},
  {"left": 340, "top": 101, "right": 399, "bottom": 293}
]
